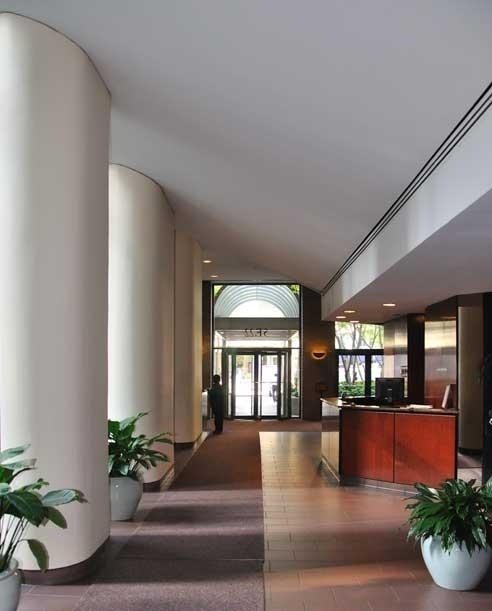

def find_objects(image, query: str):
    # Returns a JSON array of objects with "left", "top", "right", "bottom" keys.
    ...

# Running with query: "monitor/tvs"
[{"left": 376, "top": 378, "right": 404, "bottom": 408}]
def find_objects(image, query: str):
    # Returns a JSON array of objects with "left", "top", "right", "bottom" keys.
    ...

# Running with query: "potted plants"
[
  {"left": 397, "top": 474, "right": 492, "bottom": 592},
  {"left": 108, "top": 408, "right": 177, "bottom": 523},
  {"left": 1, "top": 441, "right": 82, "bottom": 610}
]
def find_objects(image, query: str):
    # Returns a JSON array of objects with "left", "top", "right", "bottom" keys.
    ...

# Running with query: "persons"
[{"left": 207, "top": 374, "right": 227, "bottom": 435}]
